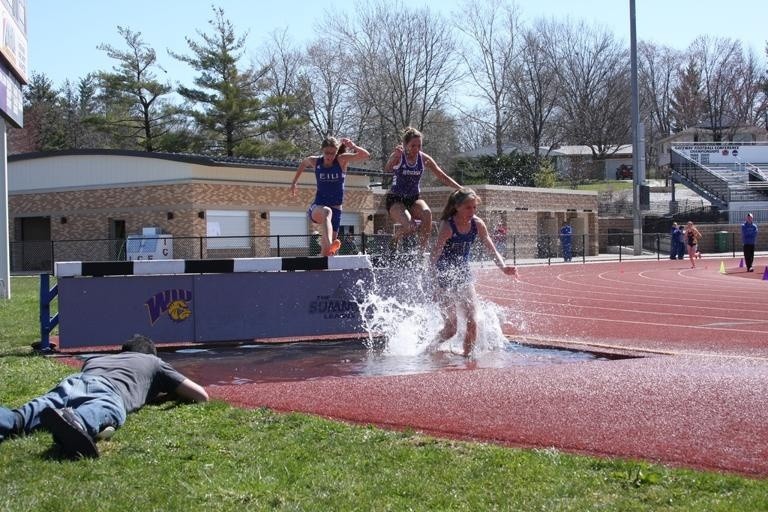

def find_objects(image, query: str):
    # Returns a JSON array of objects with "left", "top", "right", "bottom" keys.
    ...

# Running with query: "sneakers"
[
  {"left": 39, "top": 407, "right": 99, "bottom": 460},
  {"left": 748, "top": 267, "right": 754, "bottom": 272}
]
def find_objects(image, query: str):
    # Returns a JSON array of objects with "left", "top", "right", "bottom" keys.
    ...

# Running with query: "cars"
[{"left": 614, "top": 164, "right": 633, "bottom": 180}]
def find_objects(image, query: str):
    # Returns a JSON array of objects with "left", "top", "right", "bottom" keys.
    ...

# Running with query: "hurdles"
[{"left": 31, "top": 251, "right": 441, "bottom": 350}]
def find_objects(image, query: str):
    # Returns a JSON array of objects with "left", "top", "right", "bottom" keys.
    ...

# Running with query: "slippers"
[{"left": 324, "top": 239, "right": 341, "bottom": 256}]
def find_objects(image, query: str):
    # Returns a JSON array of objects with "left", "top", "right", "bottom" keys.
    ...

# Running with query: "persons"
[
  {"left": 289, "top": 136, "right": 371, "bottom": 255},
  {"left": 0, "top": 334, "right": 210, "bottom": 461},
  {"left": 678, "top": 226, "right": 686, "bottom": 259},
  {"left": 682, "top": 221, "right": 702, "bottom": 269},
  {"left": 670, "top": 222, "right": 682, "bottom": 259},
  {"left": 423, "top": 188, "right": 519, "bottom": 356},
  {"left": 310, "top": 231, "right": 322, "bottom": 256},
  {"left": 338, "top": 231, "right": 359, "bottom": 256},
  {"left": 740, "top": 212, "right": 758, "bottom": 272},
  {"left": 559, "top": 221, "right": 573, "bottom": 261},
  {"left": 490, "top": 218, "right": 508, "bottom": 261},
  {"left": 384, "top": 126, "right": 462, "bottom": 252}
]
